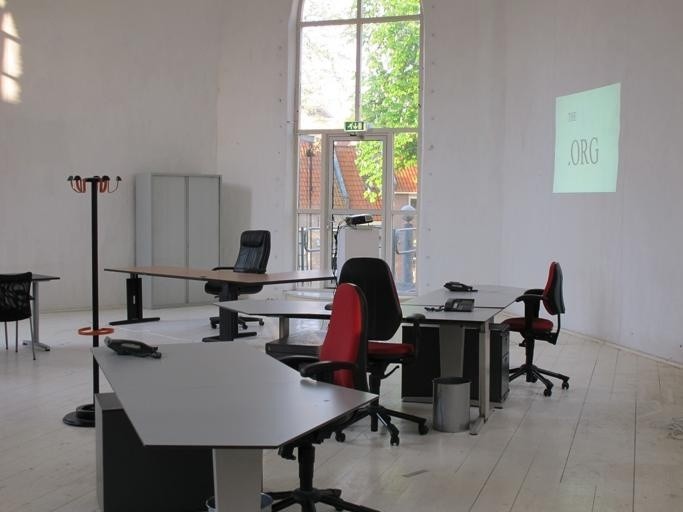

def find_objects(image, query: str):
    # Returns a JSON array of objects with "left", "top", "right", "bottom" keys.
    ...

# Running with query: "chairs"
[{"left": 0, "top": 271, "right": 37, "bottom": 360}]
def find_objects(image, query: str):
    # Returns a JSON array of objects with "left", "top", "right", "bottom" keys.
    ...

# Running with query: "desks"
[
  {"left": 0, "top": 274, "right": 60, "bottom": 352},
  {"left": 89, "top": 341, "right": 380, "bottom": 512}
]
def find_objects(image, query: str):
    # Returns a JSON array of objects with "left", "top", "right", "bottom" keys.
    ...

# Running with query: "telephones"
[
  {"left": 444, "top": 281, "right": 472, "bottom": 292},
  {"left": 444, "top": 298, "right": 475, "bottom": 312},
  {"left": 103, "top": 336, "right": 154, "bottom": 355}
]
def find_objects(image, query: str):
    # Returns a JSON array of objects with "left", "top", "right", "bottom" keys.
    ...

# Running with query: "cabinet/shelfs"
[{"left": 137, "top": 172, "right": 222, "bottom": 308}]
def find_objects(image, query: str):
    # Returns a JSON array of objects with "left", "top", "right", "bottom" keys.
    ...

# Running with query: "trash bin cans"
[{"left": 432, "top": 377, "right": 472, "bottom": 433}]
